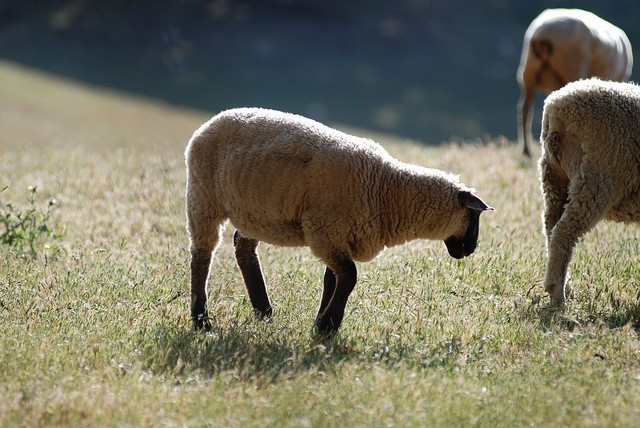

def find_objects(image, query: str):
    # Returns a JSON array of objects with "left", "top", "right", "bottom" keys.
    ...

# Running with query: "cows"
[{"left": 515, "top": 9, "right": 636, "bottom": 158}]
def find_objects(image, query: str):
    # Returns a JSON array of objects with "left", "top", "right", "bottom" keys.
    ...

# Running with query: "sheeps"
[
  {"left": 183, "top": 105, "right": 498, "bottom": 344},
  {"left": 538, "top": 77, "right": 640, "bottom": 308}
]
